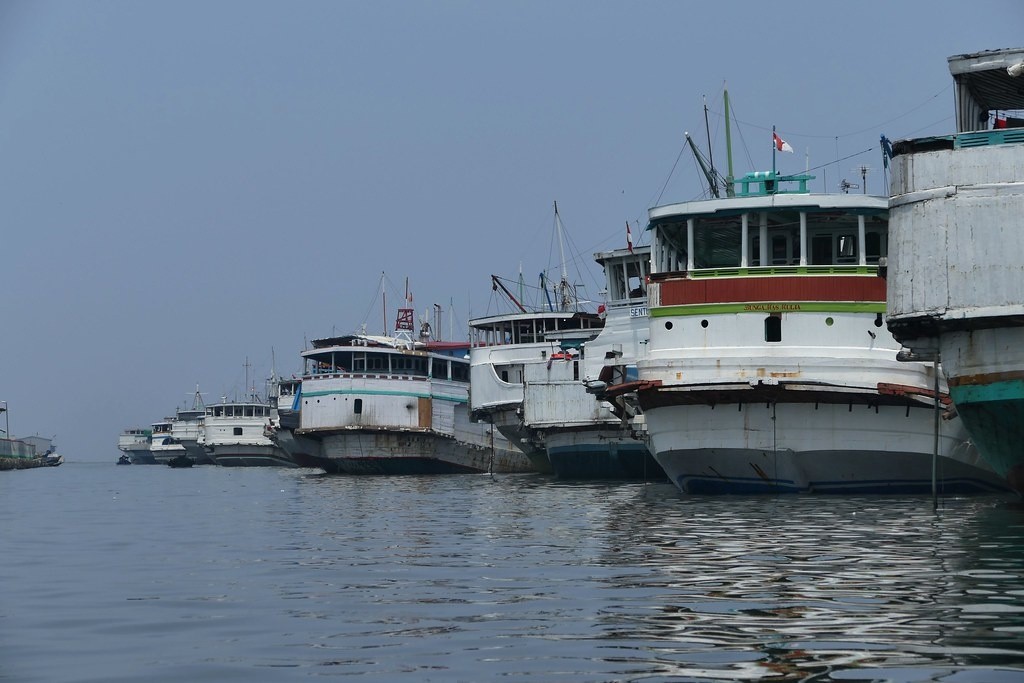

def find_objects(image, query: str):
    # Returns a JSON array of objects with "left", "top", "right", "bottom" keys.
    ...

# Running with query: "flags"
[{"left": 772, "top": 134, "right": 793, "bottom": 152}]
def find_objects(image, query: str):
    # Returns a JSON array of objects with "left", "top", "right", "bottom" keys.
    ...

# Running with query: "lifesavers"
[{"left": 552, "top": 354, "right": 572, "bottom": 359}]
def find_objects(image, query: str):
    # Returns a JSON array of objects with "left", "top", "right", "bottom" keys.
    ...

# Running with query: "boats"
[
  {"left": 118, "top": 270, "right": 553, "bottom": 475},
  {"left": 467, "top": 201, "right": 667, "bottom": 486},
  {"left": 585, "top": 79, "right": 1018, "bottom": 499},
  {"left": 886, "top": 48, "right": 1024, "bottom": 504},
  {"left": 0, "top": 401, "right": 64, "bottom": 470}
]
